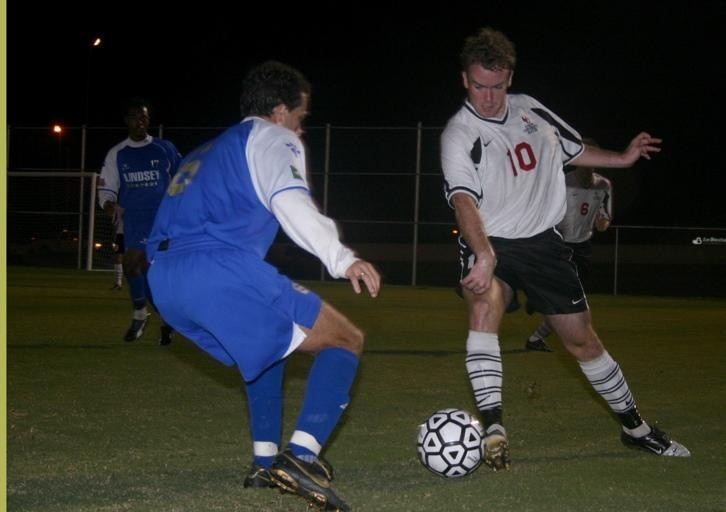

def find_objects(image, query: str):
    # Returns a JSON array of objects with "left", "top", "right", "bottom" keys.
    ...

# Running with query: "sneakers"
[
  {"left": 244, "top": 448, "right": 351, "bottom": 512},
  {"left": 525, "top": 338, "right": 553, "bottom": 352},
  {"left": 621, "top": 424, "right": 691, "bottom": 457},
  {"left": 123, "top": 313, "right": 151, "bottom": 341},
  {"left": 481, "top": 421, "right": 512, "bottom": 472},
  {"left": 160, "top": 325, "right": 174, "bottom": 346},
  {"left": 110, "top": 284, "right": 123, "bottom": 291}
]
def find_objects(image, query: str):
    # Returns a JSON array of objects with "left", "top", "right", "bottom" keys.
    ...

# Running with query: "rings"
[{"left": 359, "top": 272, "right": 365, "bottom": 279}]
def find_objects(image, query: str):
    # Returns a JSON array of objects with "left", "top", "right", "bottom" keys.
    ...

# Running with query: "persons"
[
  {"left": 97, "top": 101, "right": 184, "bottom": 346},
  {"left": 523, "top": 135, "right": 615, "bottom": 353},
  {"left": 108, "top": 201, "right": 125, "bottom": 293},
  {"left": 143, "top": 60, "right": 381, "bottom": 512},
  {"left": 438, "top": 27, "right": 691, "bottom": 472}
]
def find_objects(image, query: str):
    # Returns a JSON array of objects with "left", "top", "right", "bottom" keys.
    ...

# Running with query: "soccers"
[{"left": 415, "top": 408, "right": 485, "bottom": 478}]
